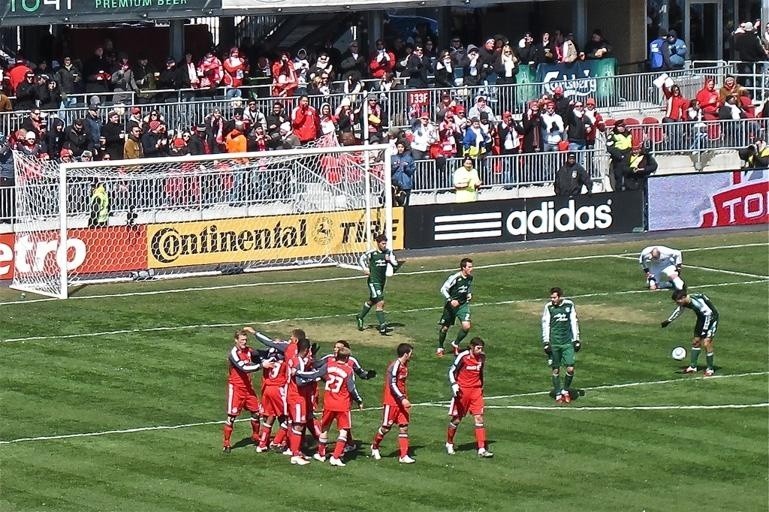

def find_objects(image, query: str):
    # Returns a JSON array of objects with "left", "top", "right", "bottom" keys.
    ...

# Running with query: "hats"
[
  {"left": 503, "top": 111, "right": 511, "bottom": 118},
  {"left": 196, "top": 123, "right": 207, "bottom": 132},
  {"left": 463, "top": 153, "right": 472, "bottom": 163},
  {"left": 612, "top": 119, "right": 627, "bottom": 127},
  {"left": 341, "top": 97, "right": 351, "bottom": 107},
  {"left": 149, "top": 120, "right": 161, "bottom": 130},
  {"left": 447, "top": 114, "right": 453, "bottom": 118},
  {"left": 25, "top": 130, "right": 36, "bottom": 141},
  {"left": 466, "top": 43, "right": 479, "bottom": 54},
  {"left": 480, "top": 111, "right": 488, "bottom": 120},
  {"left": 212, "top": 106, "right": 221, "bottom": 113},
  {"left": 420, "top": 112, "right": 429, "bottom": 119},
  {"left": 667, "top": 29, "right": 677, "bottom": 39},
  {"left": 587, "top": 98, "right": 595, "bottom": 105},
  {"left": 279, "top": 121, "right": 291, "bottom": 132},
  {"left": 568, "top": 153, "right": 575, "bottom": 158},
  {"left": 441, "top": 91, "right": 449, "bottom": 98},
  {"left": 61, "top": 149, "right": 70, "bottom": 156},
  {"left": 725, "top": 94, "right": 734, "bottom": 101},
  {"left": 657, "top": 27, "right": 667, "bottom": 36},
  {"left": 80, "top": 150, "right": 94, "bottom": 162},
  {"left": 554, "top": 86, "right": 564, "bottom": 94},
  {"left": 88, "top": 104, "right": 99, "bottom": 110}
]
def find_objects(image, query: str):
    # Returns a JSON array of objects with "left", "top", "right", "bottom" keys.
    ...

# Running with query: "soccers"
[{"left": 671, "top": 346, "right": 687, "bottom": 361}]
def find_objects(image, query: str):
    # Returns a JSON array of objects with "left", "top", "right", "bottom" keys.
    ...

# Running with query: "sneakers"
[
  {"left": 436, "top": 348, "right": 444, "bottom": 357},
  {"left": 282, "top": 448, "right": 294, "bottom": 456},
  {"left": 290, "top": 455, "right": 311, "bottom": 466},
  {"left": 562, "top": 391, "right": 571, "bottom": 403},
  {"left": 370, "top": 444, "right": 381, "bottom": 460},
  {"left": 478, "top": 449, "right": 494, "bottom": 458},
  {"left": 223, "top": 445, "right": 231, "bottom": 453},
  {"left": 299, "top": 451, "right": 312, "bottom": 460},
  {"left": 380, "top": 325, "right": 394, "bottom": 334},
  {"left": 398, "top": 454, "right": 416, "bottom": 464},
  {"left": 356, "top": 313, "right": 364, "bottom": 331},
  {"left": 251, "top": 435, "right": 261, "bottom": 443},
  {"left": 329, "top": 456, "right": 346, "bottom": 467},
  {"left": 256, "top": 445, "right": 268, "bottom": 453},
  {"left": 451, "top": 341, "right": 460, "bottom": 356},
  {"left": 281, "top": 445, "right": 289, "bottom": 451},
  {"left": 681, "top": 366, "right": 698, "bottom": 375},
  {"left": 313, "top": 454, "right": 326, "bottom": 462},
  {"left": 555, "top": 395, "right": 564, "bottom": 404},
  {"left": 343, "top": 444, "right": 357, "bottom": 453},
  {"left": 269, "top": 441, "right": 281, "bottom": 450},
  {"left": 445, "top": 442, "right": 456, "bottom": 455},
  {"left": 704, "top": 368, "right": 714, "bottom": 376}
]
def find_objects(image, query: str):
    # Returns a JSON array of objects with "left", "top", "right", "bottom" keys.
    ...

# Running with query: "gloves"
[
  {"left": 574, "top": 341, "right": 581, "bottom": 352},
  {"left": 661, "top": 320, "right": 670, "bottom": 327},
  {"left": 543, "top": 343, "right": 552, "bottom": 355},
  {"left": 366, "top": 369, "right": 377, "bottom": 379},
  {"left": 700, "top": 329, "right": 707, "bottom": 338},
  {"left": 310, "top": 342, "right": 321, "bottom": 356},
  {"left": 451, "top": 383, "right": 460, "bottom": 400}
]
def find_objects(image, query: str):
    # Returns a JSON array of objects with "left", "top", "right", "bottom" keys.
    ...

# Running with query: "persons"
[
  {"left": 446, "top": 336, "right": 493, "bottom": 457},
  {"left": 0, "top": 20, "right": 767, "bottom": 228},
  {"left": 662, "top": 291, "right": 719, "bottom": 376},
  {"left": 639, "top": 245, "right": 685, "bottom": 291},
  {"left": 542, "top": 287, "right": 581, "bottom": 404},
  {"left": 437, "top": 258, "right": 473, "bottom": 358},
  {"left": 356, "top": 234, "right": 398, "bottom": 333},
  {"left": 371, "top": 343, "right": 416, "bottom": 463},
  {"left": 223, "top": 328, "right": 378, "bottom": 471}
]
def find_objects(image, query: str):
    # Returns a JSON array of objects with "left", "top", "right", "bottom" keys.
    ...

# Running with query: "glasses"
[
  {"left": 134, "top": 111, "right": 141, "bottom": 115},
  {"left": 442, "top": 53, "right": 450, "bottom": 58},
  {"left": 377, "top": 43, "right": 384, "bottom": 45},
  {"left": 249, "top": 103, "right": 255, "bottom": 106},
  {"left": 321, "top": 58, "right": 326, "bottom": 60},
  {"left": 459, "top": 111, "right": 465, "bottom": 114},
  {"left": 182, "top": 135, "right": 190, "bottom": 138},
  {"left": 575, "top": 105, "right": 583, "bottom": 108},
  {"left": 150, "top": 114, "right": 158, "bottom": 117},
  {"left": 234, "top": 113, "right": 242, "bottom": 116},
  {"left": 32, "top": 111, "right": 40, "bottom": 115},
  {"left": 416, "top": 50, "right": 423, "bottom": 51},
  {"left": 504, "top": 51, "right": 512, "bottom": 53},
  {"left": 547, "top": 108, "right": 554, "bottom": 109},
  {"left": 103, "top": 157, "right": 111, "bottom": 160},
  {"left": 132, "top": 107, "right": 140, "bottom": 113},
  {"left": 321, "top": 77, "right": 329, "bottom": 79},
  {"left": 369, "top": 100, "right": 376, "bottom": 101},
  {"left": 587, "top": 103, "right": 594, "bottom": 106}
]
